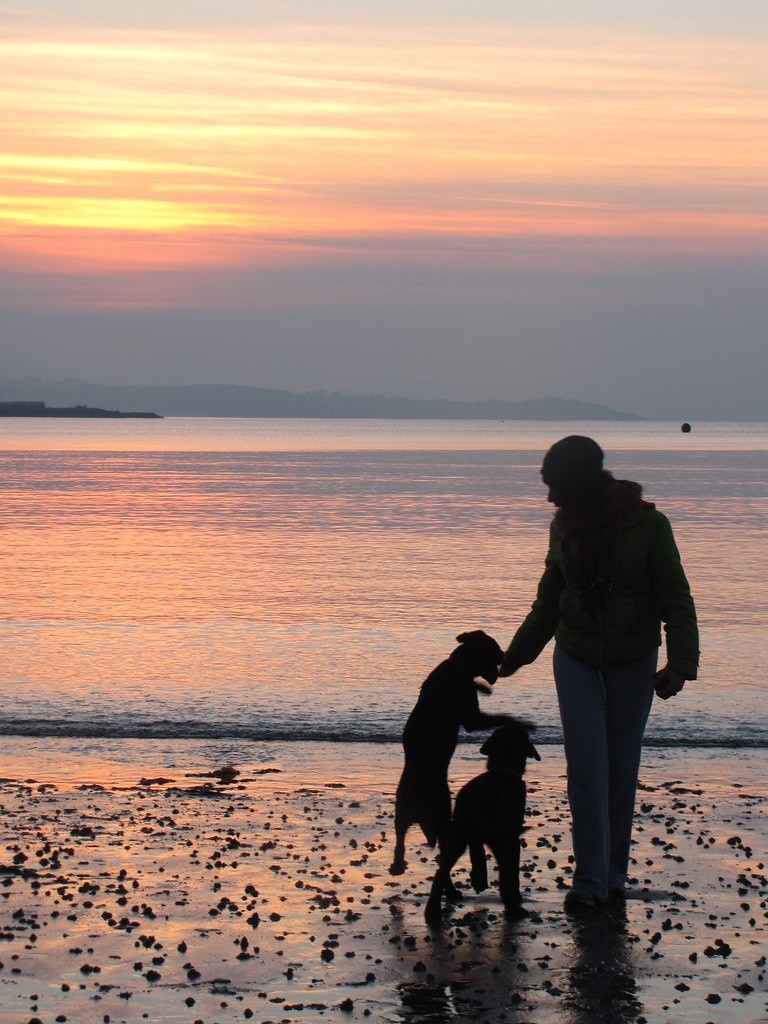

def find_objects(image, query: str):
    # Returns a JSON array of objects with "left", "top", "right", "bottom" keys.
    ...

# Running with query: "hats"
[{"left": 539, "top": 436, "right": 604, "bottom": 483}]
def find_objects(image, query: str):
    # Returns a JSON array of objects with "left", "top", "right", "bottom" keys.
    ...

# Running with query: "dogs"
[{"left": 388, "top": 630, "right": 541, "bottom": 923}]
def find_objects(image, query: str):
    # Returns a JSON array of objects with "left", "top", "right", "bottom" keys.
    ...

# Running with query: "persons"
[{"left": 498, "top": 436, "right": 701, "bottom": 929}]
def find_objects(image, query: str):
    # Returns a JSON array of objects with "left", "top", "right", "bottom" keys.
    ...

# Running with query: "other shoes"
[
  {"left": 565, "top": 888, "right": 606, "bottom": 907},
  {"left": 607, "top": 884, "right": 625, "bottom": 896}
]
